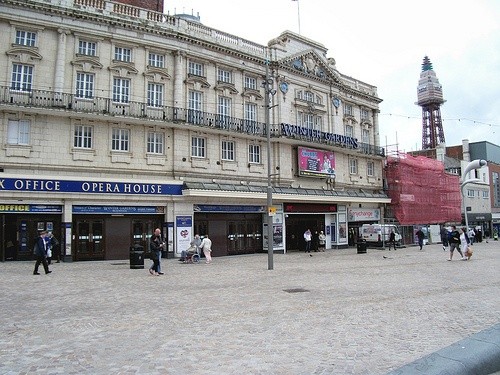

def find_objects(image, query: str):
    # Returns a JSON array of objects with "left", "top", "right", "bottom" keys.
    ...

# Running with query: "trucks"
[{"left": 361, "top": 224, "right": 403, "bottom": 247}]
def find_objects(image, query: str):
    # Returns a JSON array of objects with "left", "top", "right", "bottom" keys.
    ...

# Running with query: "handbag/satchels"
[
  {"left": 465, "top": 247, "right": 472, "bottom": 257},
  {"left": 47, "top": 248, "right": 52, "bottom": 257}
]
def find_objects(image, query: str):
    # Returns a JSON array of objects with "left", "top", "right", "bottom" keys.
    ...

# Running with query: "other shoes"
[
  {"left": 149, "top": 269, "right": 159, "bottom": 276},
  {"left": 178, "top": 259, "right": 185, "bottom": 261},
  {"left": 34, "top": 271, "right": 40, "bottom": 275},
  {"left": 158, "top": 271, "right": 164, "bottom": 274},
  {"left": 45, "top": 270, "right": 52, "bottom": 274}
]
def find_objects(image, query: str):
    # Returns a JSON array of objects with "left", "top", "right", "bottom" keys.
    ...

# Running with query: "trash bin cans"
[
  {"left": 130, "top": 245, "right": 144, "bottom": 269},
  {"left": 357, "top": 238, "right": 367, "bottom": 254}
]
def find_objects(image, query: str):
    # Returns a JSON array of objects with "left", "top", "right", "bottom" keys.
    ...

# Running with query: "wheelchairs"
[{"left": 182, "top": 245, "right": 201, "bottom": 264}]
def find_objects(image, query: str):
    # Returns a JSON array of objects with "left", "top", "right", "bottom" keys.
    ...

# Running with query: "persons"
[
  {"left": 149, "top": 228, "right": 166, "bottom": 276},
  {"left": 323, "top": 152, "right": 332, "bottom": 172},
  {"left": 389, "top": 229, "right": 396, "bottom": 251},
  {"left": 45, "top": 231, "right": 61, "bottom": 264},
  {"left": 440, "top": 224, "right": 447, "bottom": 251},
  {"left": 178, "top": 234, "right": 201, "bottom": 262},
  {"left": 33, "top": 229, "right": 52, "bottom": 275},
  {"left": 319, "top": 231, "right": 326, "bottom": 251},
  {"left": 468, "top": 228, "right": 475, "bottom": 245},
  {"left": 199, "top": 235, "right": 212, "bottom": 264},
  {"left": 415, "top": 227, "right": 424, "bottom": 250},
  {"left": 304, "top": 229, "right": 312, "bottom": 253},
  {"left": 485, "top": 229, "right": 490, "bottom": 243},
  {"left": 447, "top": 225, "right": 463, "bottom": 261},
  {"left": 460, "top": 227, "right": 470, "bottom": 261},
  {"left": 312, "top": 231, "right": 320, "bottom": 252}
]
